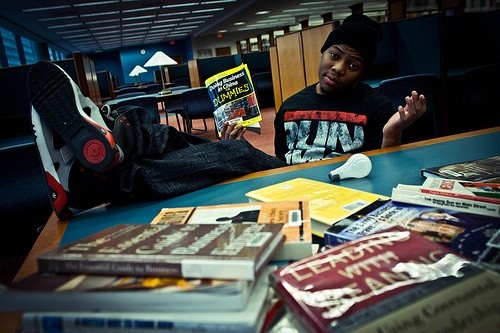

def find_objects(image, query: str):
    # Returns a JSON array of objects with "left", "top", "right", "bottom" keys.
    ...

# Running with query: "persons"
[{"left": 27, "top": 12, "right": 428, "bottom": 222}]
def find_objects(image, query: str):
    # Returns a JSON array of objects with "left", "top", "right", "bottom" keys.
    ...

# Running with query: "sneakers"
[
  {"left": 31, "top": 104, "right": 104, "bottom": 221},
  {"left": 27, "top": 61, "right": 130, "bottom": 171}
]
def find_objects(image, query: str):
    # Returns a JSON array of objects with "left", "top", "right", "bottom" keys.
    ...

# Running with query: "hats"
[{"left": 320, "top": 14, "right": 383, "bottom": 65}]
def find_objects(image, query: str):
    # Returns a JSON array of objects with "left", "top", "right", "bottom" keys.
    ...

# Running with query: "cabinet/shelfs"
[{"left": 267, "top": 20, "right": 339, "bottom": 113}]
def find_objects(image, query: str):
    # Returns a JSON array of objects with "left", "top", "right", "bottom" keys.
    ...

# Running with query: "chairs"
[
  {"left": 116, "top": 98, "right": 160, "bottom": 125},
  {"left": 456, "top": 65, "right": 499, "bottom": 126},
  {"left": 113, "top": 92, "right": 145, "bottom": 97},
  {"left": 165, "top": 87, "right": 190, "bottom": 91},
  {"left": 161, "top": 86, "right": 192, "bottom": 131},
  {"left": 375, "top": 74, "right": 439, "bottom": 138},
  {"left": 183, "top": 88, "right": 216, "bottom": 134}
]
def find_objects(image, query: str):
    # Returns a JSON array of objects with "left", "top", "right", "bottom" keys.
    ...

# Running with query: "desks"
[{"left": 0, "top": 127, "right": 500, "bottom": 333}]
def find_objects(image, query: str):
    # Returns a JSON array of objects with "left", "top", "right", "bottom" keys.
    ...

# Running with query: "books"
[
  {"left": 205, "top": 62, "right": 262, "bottom": 135},
  {"left": 0, "top": 155, "right": 500, "bottom": 332}
]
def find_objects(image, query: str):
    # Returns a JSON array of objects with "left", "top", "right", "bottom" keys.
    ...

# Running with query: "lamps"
[
  {"left": 129, "top": 65, "right": 147, "bottom": 87},
  {"left": 143, "top": 51, "right": 178, "bottom": 95}
]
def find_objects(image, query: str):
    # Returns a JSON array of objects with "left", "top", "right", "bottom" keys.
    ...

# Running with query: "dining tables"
[{"left": 104, "top": 86, "right": 207, "bottom": 132}]
